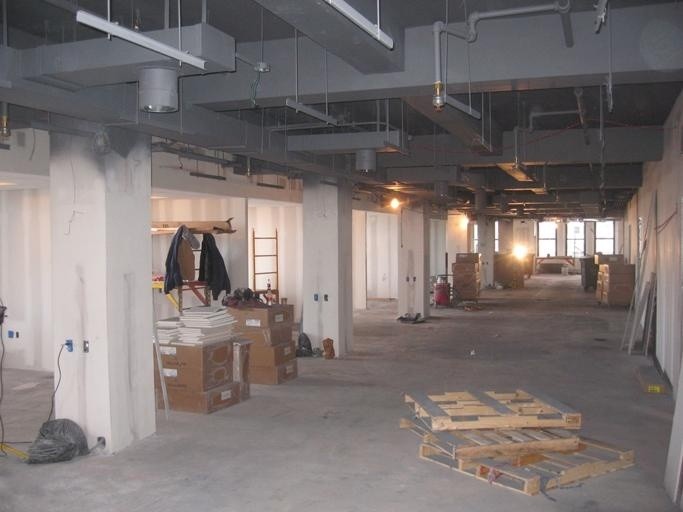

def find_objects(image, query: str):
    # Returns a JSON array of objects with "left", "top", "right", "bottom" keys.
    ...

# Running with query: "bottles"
[{"left": 266, "top": 284, "right": 272, "bottom": 306}]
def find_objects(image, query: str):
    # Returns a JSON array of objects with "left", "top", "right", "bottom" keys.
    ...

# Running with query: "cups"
[{"left": 281, "top": 298, "right": 287, "bottom": 308}]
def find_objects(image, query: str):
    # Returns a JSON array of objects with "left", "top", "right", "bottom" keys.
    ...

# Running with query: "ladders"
[{"left": 252, "top": 228, "right": 279, "bottom": 291}]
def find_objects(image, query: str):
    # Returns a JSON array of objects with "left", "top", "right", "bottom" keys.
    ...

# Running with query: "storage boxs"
[
  {"left": 594, "top": 254, "right": 635, "bottom": 304},
  {"left": 451, "top": 252, "right": 482, "bottom": 299},
  {"left": 153, "top": 303, "right": 297, "bottom": 415}
]
